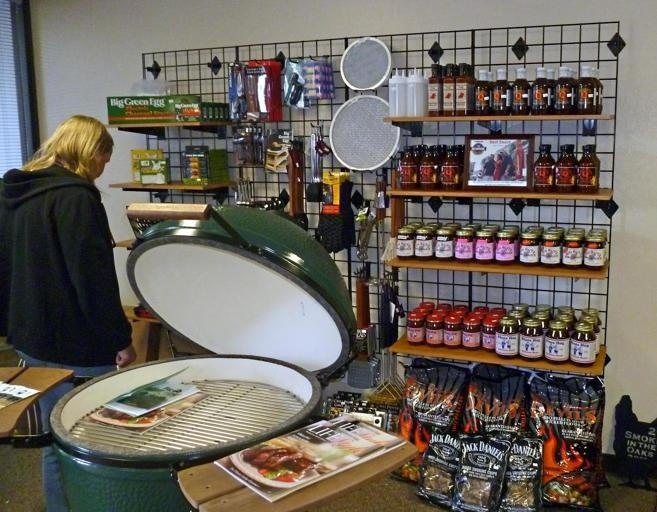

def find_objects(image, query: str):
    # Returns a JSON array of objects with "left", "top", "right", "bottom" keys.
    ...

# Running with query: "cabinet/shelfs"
[
  {"left": 104, "top": 116, "right": 238, "bottom": 330},
  {"left": 380, "top": 109, "right": 619, "bottom": 385}
]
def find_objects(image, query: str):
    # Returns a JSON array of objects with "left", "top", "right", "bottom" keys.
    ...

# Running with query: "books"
[
  {"left": 329, "top": 414, "right": 410, "bottom": 452},
  {"left": 103, "top": 368, "right": 195, "bottom": 417},
  {"left": 90, "top": 391, "right": 209, "bottom": 435},
  {"left": 213, "top": 421, "right": 382, "bottom": 502}
]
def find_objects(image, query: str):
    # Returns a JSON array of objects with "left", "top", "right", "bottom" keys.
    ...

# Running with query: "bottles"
[
  {"left": 397, "top": 142, "right": 465, "bottom": 189},
  {"left": 532, "top": 142, "right": 600, "bottom": 190},
  {"left": 386, "top": 62, "right": 602, "bottom": 118}
]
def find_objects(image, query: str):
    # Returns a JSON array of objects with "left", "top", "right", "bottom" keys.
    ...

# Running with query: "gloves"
[{"left": 315, "top": 172, "right": 355, "bottom": 253}]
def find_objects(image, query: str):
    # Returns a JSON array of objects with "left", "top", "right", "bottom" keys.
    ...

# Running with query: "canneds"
[
  {"left": 407, "top": 301, "right": 602, "bottom": 367},
  {"left": 397, "top": 223, "right": 607, "bottom": 271}
]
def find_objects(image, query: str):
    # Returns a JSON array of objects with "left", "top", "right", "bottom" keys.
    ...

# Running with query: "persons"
[
  {"left": 476, "top": 151, "right": 514, "bottom": 181},
  {"left": 0, "top": 116, "right": 137, "bottom": 511}
]
